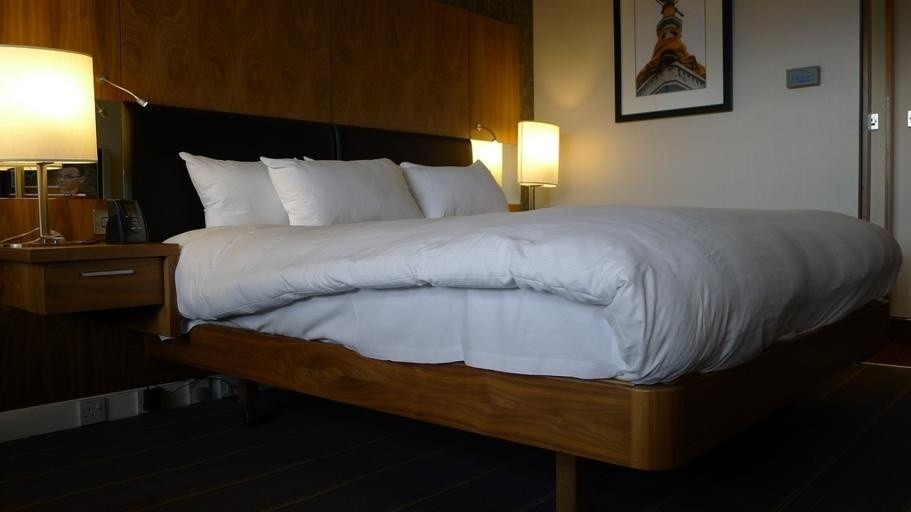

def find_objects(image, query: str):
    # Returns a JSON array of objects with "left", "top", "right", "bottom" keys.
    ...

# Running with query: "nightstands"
[{"left": 1, "top": 234, "right": 185, "bottom": 345}]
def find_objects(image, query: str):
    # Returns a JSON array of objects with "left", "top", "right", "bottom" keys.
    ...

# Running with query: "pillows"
[
  {"left": 398, "top": 158, "right": 515, "bottom": 221},
  {"left": 176, "top": 149, "right": 310, "bottom": 230},
  {"left": 257, "top": 150, "right": 427, "bottom": 228}
]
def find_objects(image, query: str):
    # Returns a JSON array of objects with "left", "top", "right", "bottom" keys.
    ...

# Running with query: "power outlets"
[
  {"left": 135, "top": 383, "right": 175, "bottom": 416},
  {"left": 210, "top": 370, "right": 243, "bottom": 401},
  {"left": 184, "top": 374, "right": 213, "bottom": 406},
  {"left": 75, "top": 397, "right": 108, "bottom": 426}
]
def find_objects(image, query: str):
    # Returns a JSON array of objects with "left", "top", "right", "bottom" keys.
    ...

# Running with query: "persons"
[{"left": 51, "top": 165, "right": 94, "bottom": 197}]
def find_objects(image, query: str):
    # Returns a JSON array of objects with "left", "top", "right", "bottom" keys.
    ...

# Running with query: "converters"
[{"left": 143, "top": 387, "right": 167, "bottom": 410}]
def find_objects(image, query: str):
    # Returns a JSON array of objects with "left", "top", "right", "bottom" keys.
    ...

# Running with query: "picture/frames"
[{"left": 609, "top": 0, "right": 737, "bottom": 123}]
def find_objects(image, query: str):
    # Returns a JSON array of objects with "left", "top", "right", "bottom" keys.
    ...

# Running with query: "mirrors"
[
  {"left": 1, "top": 96, "right": 129, "bottom": 203},
  {"left": 468, "top": 135, "right": 525, "bottom": 210}
]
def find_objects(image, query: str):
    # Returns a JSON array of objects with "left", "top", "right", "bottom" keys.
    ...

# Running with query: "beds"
[{"left": 111, "top": 100, "right": 903, "bottom": 510}]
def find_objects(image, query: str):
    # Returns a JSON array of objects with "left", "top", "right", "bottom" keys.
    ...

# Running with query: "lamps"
[
  {"left": 475, "top": 122, "right": 500, "bottom": 144},
  {"left": 469, "top": 137, "right": 505, "bottom": 191},
  {"left": 1, "top": 39, "right": 102, "bottom": 246},
  {"left": 511, "top": 119, "right": 562, "bottom": 210},
  {"left": 95, "top": 70, "right": 150, "bottom": 110}
]
